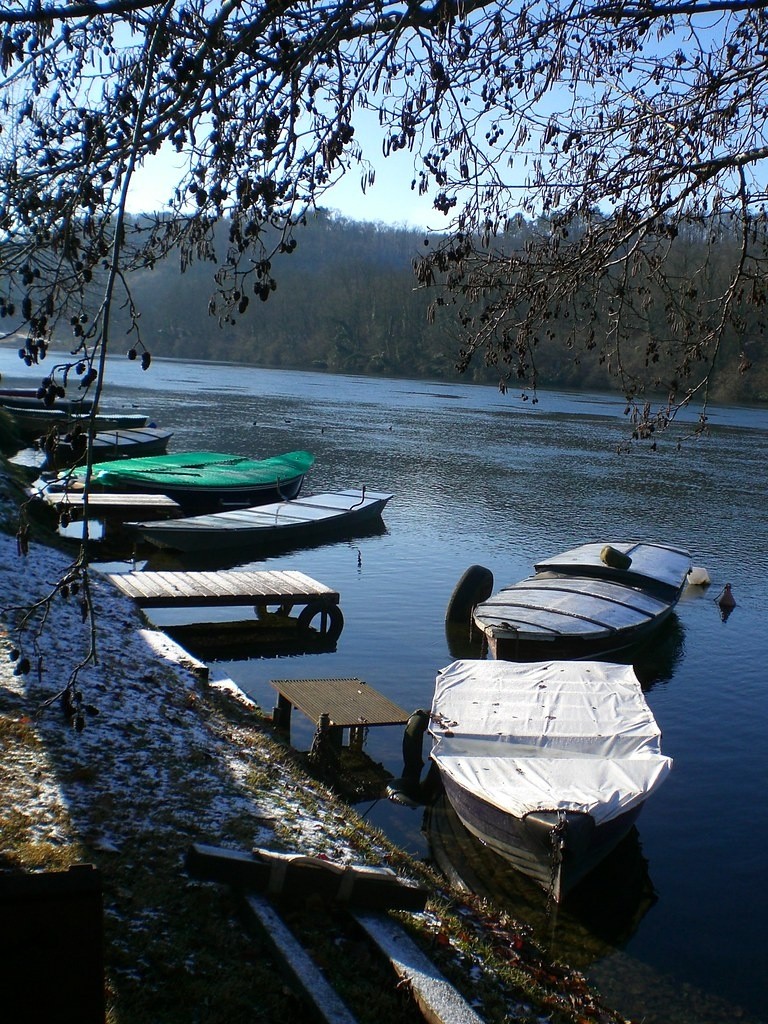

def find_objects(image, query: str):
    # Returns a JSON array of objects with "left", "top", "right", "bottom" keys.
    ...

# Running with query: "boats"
[
  {"left": 0, "top": 385, "right": 174, "bottom": 464},
  {"left": 472, "top": 539, "right": 692, "bottom": 661},
  {"left": 57, "top": 449, "right": 316, "bottom": 513},
  {"left": 426, "top": 658, "right": 674, "bottom": 885},
  {"left": 122, "top": 487, "right": 396, "bottom": 554}
]
